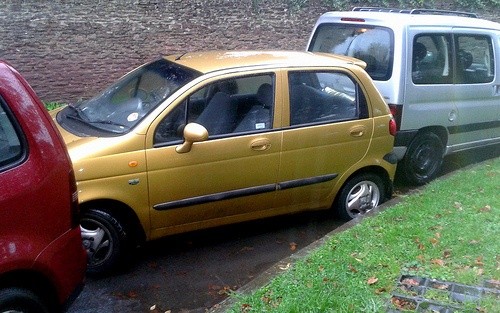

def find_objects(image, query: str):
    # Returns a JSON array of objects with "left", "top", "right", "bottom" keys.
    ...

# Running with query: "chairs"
[
  {"left": 460, "top": 49, "right": 482, "bottom": 83},
  {"left": 413, "top": 42, "right": 433, "bottom": 84},
  {"left": 366, "top": 41, "right": 388, "bottom": 74},
  {"left": 233, "top": 83, "right": 273, "bottom": 133},
  {"left": 195, "top": 78, "right": 239, "bottom": 136}
]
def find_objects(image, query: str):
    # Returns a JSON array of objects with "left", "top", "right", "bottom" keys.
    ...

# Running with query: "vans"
[{"left": 305, "top": 7, "right": 499, "bottom": 187}]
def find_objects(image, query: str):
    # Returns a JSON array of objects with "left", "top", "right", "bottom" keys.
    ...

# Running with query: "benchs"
[{"left": 290, "top": 85, "right": 353, "bottom": 125}]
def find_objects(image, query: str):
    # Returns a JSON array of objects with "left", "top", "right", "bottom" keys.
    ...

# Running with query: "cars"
[{"left": 48, "top": 49, "right": 397, "bottom": 282}]
